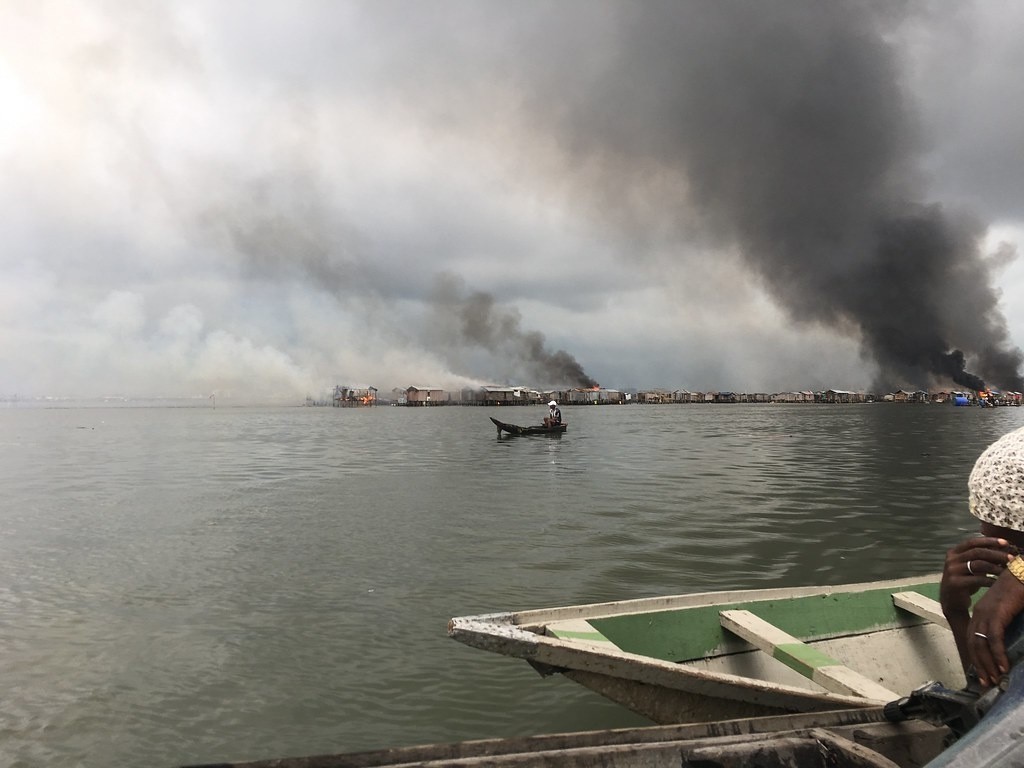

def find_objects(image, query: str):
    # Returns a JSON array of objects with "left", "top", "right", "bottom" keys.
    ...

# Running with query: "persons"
[
  {"left": 938, "top": 425, "right": 1024, "bottom": 689},
  {"left": 544, "top": 401, "right": 561, "bottom": 428}
]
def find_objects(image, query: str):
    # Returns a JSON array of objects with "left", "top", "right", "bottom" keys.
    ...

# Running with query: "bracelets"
[{"left": 1007, "top": 555, "right": 1024, "bottom": 582}]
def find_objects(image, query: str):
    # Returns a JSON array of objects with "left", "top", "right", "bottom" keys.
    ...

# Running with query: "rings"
[
  {"left": 974, "top": 632, "right": 987, "bottom": 639},
  {"left": 966, "top": 560, "right": 974, "bottom": 574}
]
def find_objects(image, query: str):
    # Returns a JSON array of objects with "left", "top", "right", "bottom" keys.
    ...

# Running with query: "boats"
[{"left": 490, "top": 416, "right": 568, "bottom": 435}]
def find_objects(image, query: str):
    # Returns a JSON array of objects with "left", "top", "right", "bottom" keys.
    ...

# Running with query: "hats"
[
  {"left": 968, "top": 426, "right": 1024, "bottom": 531},
  {"left": 548, "top": 401, "right": 556, "bottom": 406}
]
